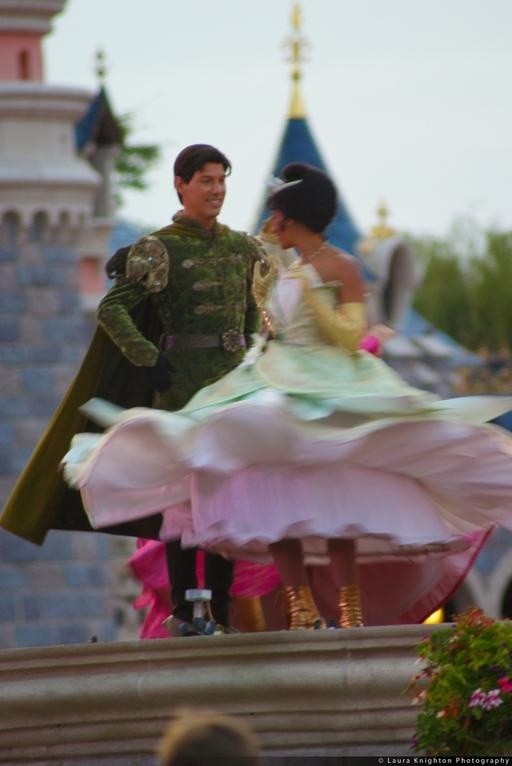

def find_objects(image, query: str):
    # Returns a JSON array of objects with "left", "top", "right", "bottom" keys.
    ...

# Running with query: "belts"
[{"left": 165, "top": 328, "right": 245, "bottom": 352}]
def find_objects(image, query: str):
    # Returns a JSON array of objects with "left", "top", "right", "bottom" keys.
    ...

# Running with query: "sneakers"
[{"left": 165, "top": 615, "right": 241, "bottom": 634}]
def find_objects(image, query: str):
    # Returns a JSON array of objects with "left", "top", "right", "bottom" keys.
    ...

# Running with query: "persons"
[
  {"left": 55, "top": 161, "right": 512, "bottom": 630},
  {"left": 0, "top": 143, "right": 275, "bottom": 637},
  {"left": 154, "top": 711, "right": 262, "bottom": 766}
]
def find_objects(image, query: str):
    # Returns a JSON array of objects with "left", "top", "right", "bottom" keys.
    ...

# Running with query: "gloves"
[
  {"left": 143, "top": 356, "right": 171, "bottom": 394},
  {"left": 251, "top": 261, "right": 280, "bottom": 338},
  {"left": 303, "top": 275, "right": 368, "bottom": 347}
]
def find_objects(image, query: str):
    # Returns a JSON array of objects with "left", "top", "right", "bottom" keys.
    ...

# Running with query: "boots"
[
  {"left": 337, "top": 585, "right": 362, "bottom": 626},
  {"left": 285, "top": 586, "right": 325, "bottom": 629}
]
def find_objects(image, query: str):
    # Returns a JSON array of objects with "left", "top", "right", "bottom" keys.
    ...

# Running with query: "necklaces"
[{"left": 302, "top": 240, "right": 328, "bottom": 262}]
